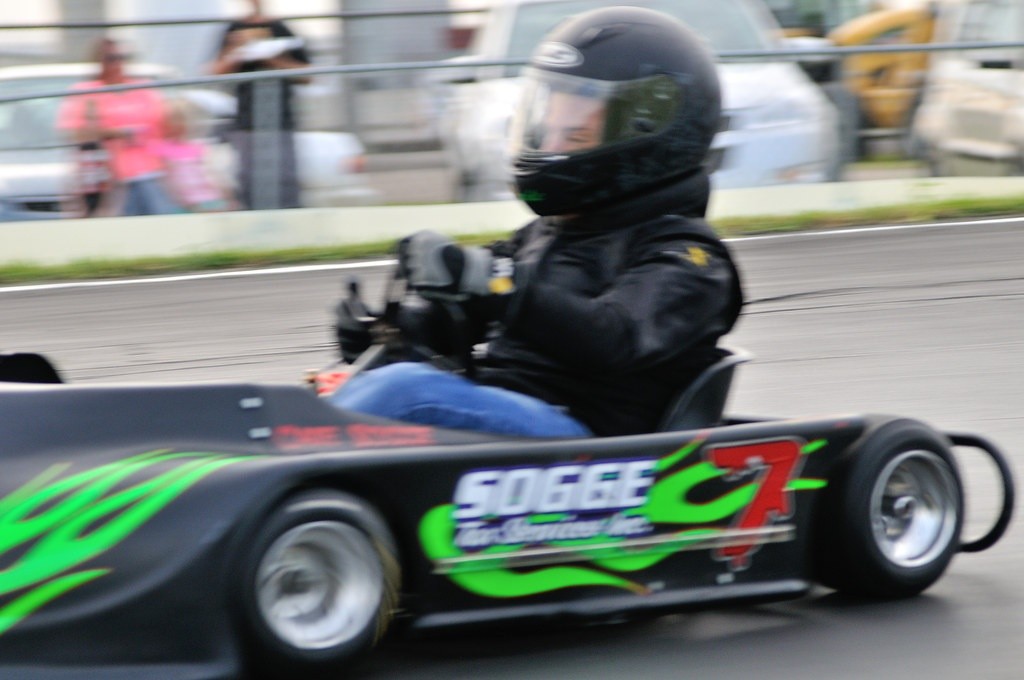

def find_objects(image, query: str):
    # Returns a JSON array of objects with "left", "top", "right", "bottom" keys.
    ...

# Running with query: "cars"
[{"left": 1, "top": 2, "right": 1024, "bottom": 224}]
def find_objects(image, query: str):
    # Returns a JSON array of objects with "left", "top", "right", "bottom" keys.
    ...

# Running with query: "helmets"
[{"left": 508, "top": 5, "right": 722, "bottom": 216}]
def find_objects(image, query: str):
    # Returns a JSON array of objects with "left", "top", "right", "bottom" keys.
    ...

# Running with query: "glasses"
[{"left": 103, "top": 52, "right": 128, "bottom": 62}]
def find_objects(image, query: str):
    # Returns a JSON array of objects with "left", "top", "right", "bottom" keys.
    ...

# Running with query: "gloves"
[
  {"left": 332, "top": 277, "right": 386, "bottom": 364},
  {"left": 399, "top": 230, "right": 514, "bottom": 322}
]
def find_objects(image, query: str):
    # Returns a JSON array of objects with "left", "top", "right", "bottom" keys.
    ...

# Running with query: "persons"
[
  {"left": 198, "top": 0, "right": 317, "bottom": 212},
  {"left": 57, "top": 34, "right": 190, "bottom": 219},
  {"left": 339, "top": 7, "right": 745, "bottom": 440}
]
